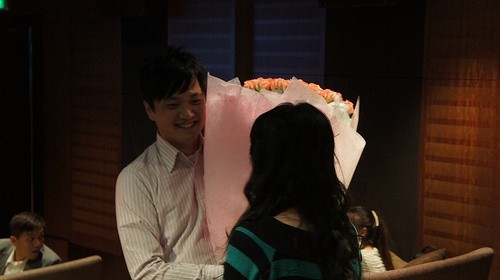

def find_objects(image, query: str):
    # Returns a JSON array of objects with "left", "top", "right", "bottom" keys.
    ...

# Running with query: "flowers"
[{"left": 244, "top": 76, "right": 356, "bottom": 117}]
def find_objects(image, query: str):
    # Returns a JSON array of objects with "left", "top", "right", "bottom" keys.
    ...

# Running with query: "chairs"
[
  {"left": 0, "top": 253, "right": 104, "bottom": 280},
  {"left": 362, "top": 247, "right": 499, "bottom": 280}
]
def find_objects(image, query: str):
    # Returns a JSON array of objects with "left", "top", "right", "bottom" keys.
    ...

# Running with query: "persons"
[
  {"left": 115, "top": 45, "right": 225, "bottom": 280},
  {"left": 223, "top": 102, "right": 363, "bottom": 280},
  {"left": 0, "top": 212, "right": 62, "bottom": 276},
  {"left": 346, "top": 206, "right": 395, "bottom": 275}
]
